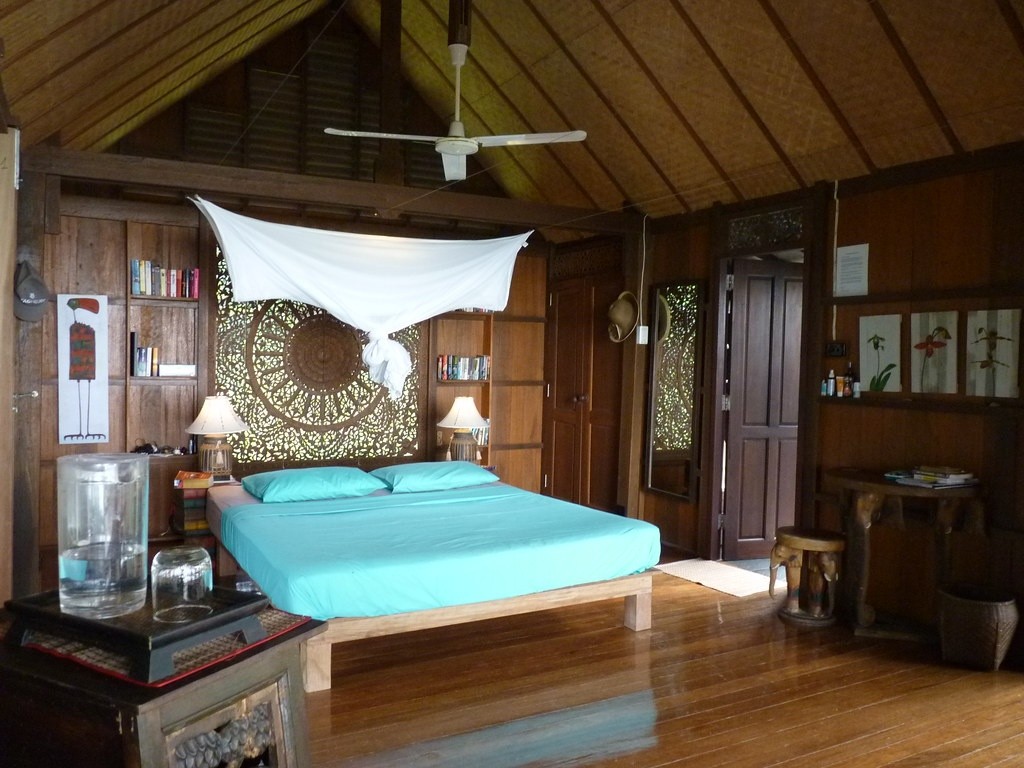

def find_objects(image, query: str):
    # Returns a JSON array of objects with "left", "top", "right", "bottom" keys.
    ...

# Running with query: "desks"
[
  {"left": 821, "top": 463, "right": 990, "bottom": 644},
  {"left": 0, "top": 607, "right": 331, "bottom": 768}
]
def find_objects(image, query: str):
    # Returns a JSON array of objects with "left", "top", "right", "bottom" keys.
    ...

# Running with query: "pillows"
[
  {"left": 241, "top": 466, "right": 389, "bottom": 507},
  {"left": 368, "top": 459, "right": 502, "bottom": 494}
]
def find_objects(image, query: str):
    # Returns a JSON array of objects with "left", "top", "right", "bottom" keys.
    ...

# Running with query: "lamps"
[
  {"left": 435, "top": 396, "right": 491, "bottom": 464},
  {"left": 185, "top": 395, "right": 247, "bottom": 476}
]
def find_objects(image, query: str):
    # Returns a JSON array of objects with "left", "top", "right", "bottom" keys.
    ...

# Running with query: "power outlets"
[
  {"left": 436, "top": 430, "right": 443, "bottom": 447},
  {"left": 824, "top": 338, "right": 849, "bottom": 361}
]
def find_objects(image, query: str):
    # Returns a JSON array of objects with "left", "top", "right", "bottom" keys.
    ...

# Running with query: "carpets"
[{"left": 653, "top": 558, "right": 789, "bottom": 598}]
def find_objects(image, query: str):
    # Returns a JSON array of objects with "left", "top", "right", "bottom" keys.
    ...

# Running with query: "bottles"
[{"left": 820, "top": 360, "right": 861, "bottom": 398}]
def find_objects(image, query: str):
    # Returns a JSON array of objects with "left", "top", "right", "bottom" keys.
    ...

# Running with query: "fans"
[{"left": 324, "top": 0, "right": 588, "bottom": 182}]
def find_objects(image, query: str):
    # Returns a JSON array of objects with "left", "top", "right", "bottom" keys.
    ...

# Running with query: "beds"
[{"left": 206, "top": 453, "right": 662, "bottom": 690}]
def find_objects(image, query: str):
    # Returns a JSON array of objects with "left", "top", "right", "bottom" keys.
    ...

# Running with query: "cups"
[
  {"left": 149, "top": 545, "right": 217, "bottom": 624},
  {"left": 55, "top": 451, "right": 150, "bottom": 621}
]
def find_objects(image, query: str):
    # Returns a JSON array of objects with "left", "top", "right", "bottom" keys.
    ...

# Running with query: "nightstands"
[{"left": 168, "top": 472, "right": 243, "bottom": 574}]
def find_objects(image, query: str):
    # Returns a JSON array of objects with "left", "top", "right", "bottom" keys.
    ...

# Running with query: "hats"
[
  {"left": 658, "top": 295, "right": 670, "bottom": 342},
  {"left": 13, "top": 260, "right": 49, "bottom": 322},
  {"left": 608, "top": 291, "right": 639, "bottom": 343}
]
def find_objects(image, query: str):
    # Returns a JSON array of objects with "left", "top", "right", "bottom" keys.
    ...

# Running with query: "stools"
[{"left": 770, "top": 524, "right": 848, "bottom": 631}]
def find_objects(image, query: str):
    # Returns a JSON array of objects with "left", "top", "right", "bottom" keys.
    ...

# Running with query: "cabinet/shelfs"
[
  {"left": 424, "top": 243, "right": 550, "bottom": 499},
  {"left": 38, "top": 188, "right": 209, "bottom": 596}
]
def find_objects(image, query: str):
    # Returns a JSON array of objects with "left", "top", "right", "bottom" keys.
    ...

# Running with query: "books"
[
  {"left": 472, "top": 418, "right": 490, "bottom": 445},
  {"left": 884, "top": 464, "right": 979, "bottom": 489},
  {"left": 130, "top": 332, "right": 158, "bottom": 376},
  {"left": 132, "top": 259, "right": 199, "bottom": 299},
  {"left": 437, "top": 354, "right": 490, "bottom": 380},
  {"left": 174, "top": 470, "right": 214, "bottom": 488}
]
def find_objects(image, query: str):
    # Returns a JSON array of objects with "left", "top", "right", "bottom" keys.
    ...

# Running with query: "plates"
[{"left": 884, "top": 470, "right": 914, "bottom": 479}]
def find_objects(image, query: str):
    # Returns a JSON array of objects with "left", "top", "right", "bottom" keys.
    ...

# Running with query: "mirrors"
[{"left": 641, "top": 278, "right": 706, "bottom": 505}]
